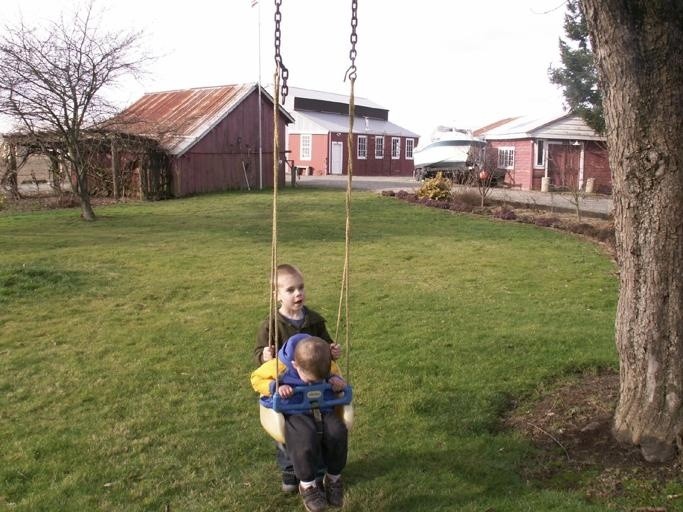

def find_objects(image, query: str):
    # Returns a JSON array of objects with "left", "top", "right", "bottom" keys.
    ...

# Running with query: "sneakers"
[
  {"left": 298, "top": 482, "right": 327, "bottom": 512},
  {"left": 281, "top": 465, "right": 299, "bottom": 492},
  {"left": 322, "top": 474, "right": 344, "bottom": 509}
]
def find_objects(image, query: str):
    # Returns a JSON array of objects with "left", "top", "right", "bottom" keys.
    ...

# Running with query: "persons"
[
  {"left": 248, "top": 333, "right": 346, "bottom": 512},
  {"left": 253, "top": 264, "right": 342, "bottom": 494}
]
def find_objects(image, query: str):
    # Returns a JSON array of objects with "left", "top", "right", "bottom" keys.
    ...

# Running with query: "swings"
[{"left": 259, "top": 1, "right": 358, "bottom": 443}]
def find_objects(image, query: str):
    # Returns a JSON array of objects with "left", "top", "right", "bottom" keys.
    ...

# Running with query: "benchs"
[{"left": 293, "top": 165, "right": 313, "bottom": 175}]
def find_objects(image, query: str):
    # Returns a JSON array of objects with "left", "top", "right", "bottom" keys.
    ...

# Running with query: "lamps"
[{"left": 571, "top": 139, "right": 583, "bottom": 147}]
